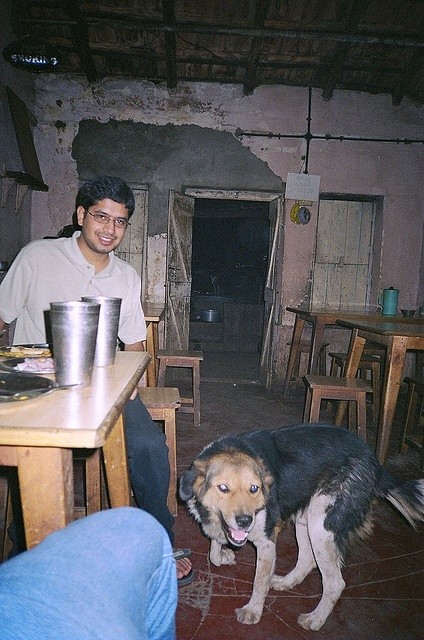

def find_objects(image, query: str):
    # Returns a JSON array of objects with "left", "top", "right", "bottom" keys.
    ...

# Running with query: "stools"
[
  {"left": 328, "top": 353, "right": 381, "bottom": 433},
  {"left": 134, "top": 385, "right": 181, "bottom": 516},
  {"left": 155, "top": 348, "right": 205, "bottom": 426},
  {"left": 2, "top": 447, "right": 104, "bottom": 564},
  {"left": 287, "top": 339, "right": 330, "bottom": 390},
  {"left": 399, "top": 374, "right": 424, "bottom": 469},
  {"left": 301, "top": 375, "right": 372, "bottom": 445}
]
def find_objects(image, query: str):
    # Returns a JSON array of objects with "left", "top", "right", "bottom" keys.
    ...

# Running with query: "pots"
[{"left": 199, "top": 309, "right": 221, "bottom": 323}]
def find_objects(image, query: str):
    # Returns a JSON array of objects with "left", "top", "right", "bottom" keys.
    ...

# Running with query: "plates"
[
  {"left": 0, "top": 343, "right": 53, "bottom": 358},
  {"left": 0, "top": 372, "right": 59, "bottom": 405},
  {"left": 0, "top": 357, "right": 56, "bottom": 376}
]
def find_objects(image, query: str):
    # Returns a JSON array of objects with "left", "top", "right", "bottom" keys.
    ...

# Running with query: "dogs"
[{"left": 178, "top": 424, "right": 424, "bottom": 632}]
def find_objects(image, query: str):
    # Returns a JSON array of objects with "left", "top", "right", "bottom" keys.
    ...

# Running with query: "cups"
[
  {"left": 81, "top": 297, "right": 121, "bottom": 368},
  {"left": 48, "top": 301, "right": 100, "bottom": 391}
]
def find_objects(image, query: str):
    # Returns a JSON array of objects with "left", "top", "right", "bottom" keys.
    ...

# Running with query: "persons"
[
  {"left": 0, "top": 174, "right": 196, "bottom": 589},
  {"left": 0, "top": 506, "right": 180, "bottom": 640}
]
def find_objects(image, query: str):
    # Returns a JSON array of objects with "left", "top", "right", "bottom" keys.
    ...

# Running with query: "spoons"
[{"left": 0, "top": 383, "right": 77, "bottom": 400}]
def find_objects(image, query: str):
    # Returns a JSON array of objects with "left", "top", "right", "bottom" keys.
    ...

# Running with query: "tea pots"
[{"left": 377, "top": 287, "right": 399, "bottom": 318}]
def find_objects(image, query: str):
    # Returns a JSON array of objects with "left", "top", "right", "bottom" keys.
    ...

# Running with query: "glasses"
[{"left": 88, "top": 212, "right": 131, "bottom": 228}]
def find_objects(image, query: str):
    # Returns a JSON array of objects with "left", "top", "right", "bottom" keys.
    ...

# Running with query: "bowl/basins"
[{"left": 400, "top": 309, "right": 415, "bottom": 317}]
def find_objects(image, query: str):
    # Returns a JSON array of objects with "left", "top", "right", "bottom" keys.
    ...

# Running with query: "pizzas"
[{"left": 1, "top": 345, "right": 53, "bottom": 358}]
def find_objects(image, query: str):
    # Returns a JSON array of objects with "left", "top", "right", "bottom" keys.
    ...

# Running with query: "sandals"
[{"left": 174, "top": 549, "right": 195, "bottom": 587}]
